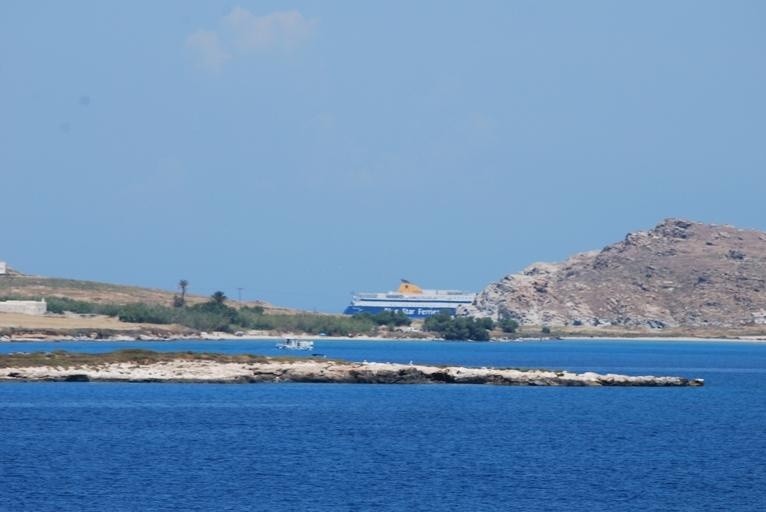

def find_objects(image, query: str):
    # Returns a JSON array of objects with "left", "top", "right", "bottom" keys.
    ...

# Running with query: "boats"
[{"left": 274, "top": 338, "right": 315, "bottom": 353}]
